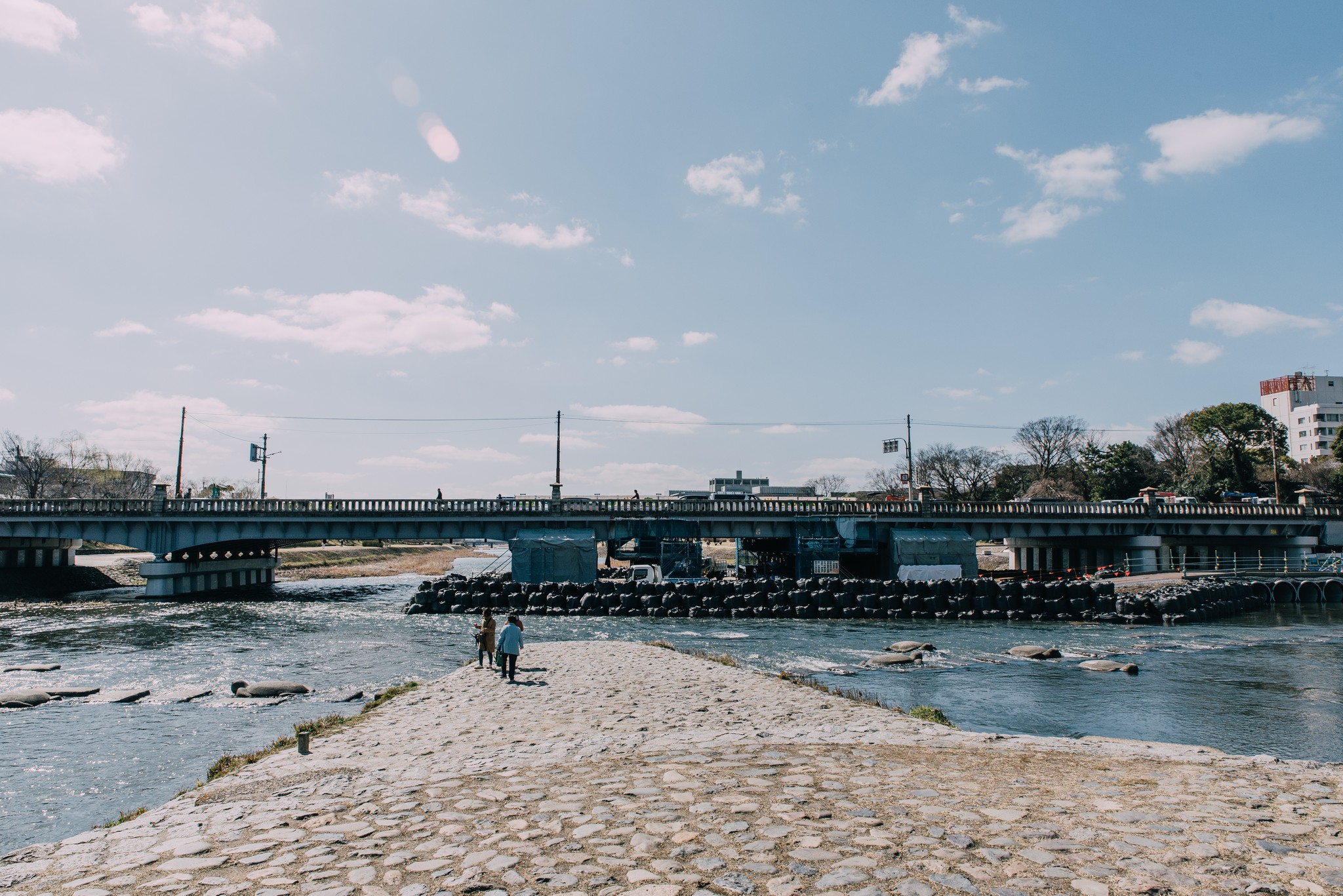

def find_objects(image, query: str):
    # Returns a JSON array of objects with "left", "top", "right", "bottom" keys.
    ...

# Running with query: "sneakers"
[
  {"left": 500, "top": 675, "right": 507, "bottom": 679},
  {"left": 486, "top": 664, "right": 492, "bottom": 668},
  {"left": 474, "top": 665, "right": 484, "bottom": 669},
  {"left": 510, "top": 678, "right": 514, "bottom": 682}
]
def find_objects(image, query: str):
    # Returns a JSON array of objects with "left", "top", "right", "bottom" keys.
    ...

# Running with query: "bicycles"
[
  {"left": 622, "top": 498, "right": 644, "bottom": 511},
  {"left": 493, "top": 501, "right": 509, "bottom": 511}
]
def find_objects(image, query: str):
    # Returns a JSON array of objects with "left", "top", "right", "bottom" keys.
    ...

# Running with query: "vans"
[
  {"left": 1101, "top": 500, "right": 1127, "bottom": 513},
  {"left": 1169, "top": 497, "right": 1197, "bottom": 513},
  {"left": 709, "top": 493, "right": 765, "bottom": 512},
  {"left": 560, "top": 497, "right": 607, "bottom": 512},
  {"left": 1020, "top": 498, "right": 1071, "bottom": 513},
  {"left": 1114, "top": 497, "right": 1166, "bottom": 514}
]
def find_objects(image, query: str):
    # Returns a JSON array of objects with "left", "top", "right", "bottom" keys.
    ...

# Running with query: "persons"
[
  {"left": 504, "top": 611, "right": 524, "bottom": 669},
  {"left": 631, "top": 489, "right": 640, "bottom": 508},
  {"left": 1013, "top": 495, "right": 1020, "bottom": 506},
  {"left": 496, "top": 616, "right": 524, "bottom": 682},
  {"left": 436, "top": 489, "right": 444, "bottom": 510},
  {"left": 495, "top": 493, "right": 503, "bottom": 508},
  {"left": 706, "top": 540, "right": 711, "bottom": 547},
  {"left": 184, "top": 488, "right": 192, "bottom": 511},
  {"left": 473, "top": 608, "right": 496, "bottom": 670},
  {"left": 176, "top": 490, "right": 184, "bottom": 505}
]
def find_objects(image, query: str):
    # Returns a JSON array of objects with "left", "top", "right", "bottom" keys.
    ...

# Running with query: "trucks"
[
  {"left": 701, "top": 557, "right": 727, "bottom": 581},
  {"left": 627, "top": 564, "right": 709, "bottom": 585}
]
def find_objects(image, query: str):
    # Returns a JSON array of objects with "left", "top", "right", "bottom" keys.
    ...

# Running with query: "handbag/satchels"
[
  {"left": 471, "top": 631, "right": 486, "bottom": 650},
  {"left": 493, "top": 649, "right": 503, "bottom": 667}
]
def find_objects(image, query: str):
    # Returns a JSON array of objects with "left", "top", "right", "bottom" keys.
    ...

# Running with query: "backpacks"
[{"left": 183, "top": 492, "right": 189, "bottom": 499}]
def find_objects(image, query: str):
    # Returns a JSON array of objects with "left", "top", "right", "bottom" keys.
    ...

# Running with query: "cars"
[
  {"left": 665, "top": 495, "right": 710, "bottom": 511},
  {"left": 441, "top": 499, "right": 487, "bottom": 511}
]
